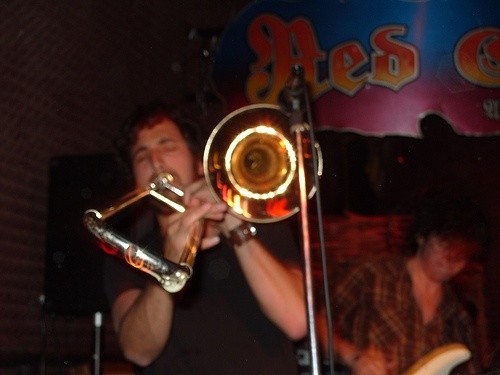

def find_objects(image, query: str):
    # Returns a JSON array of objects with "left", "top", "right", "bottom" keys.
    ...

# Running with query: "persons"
[
  {"left": 97, "top": 101, "right": 310, "bottom": 375},
  {"left": 313, "top": 199, "right": 500, "bottom": 375}
]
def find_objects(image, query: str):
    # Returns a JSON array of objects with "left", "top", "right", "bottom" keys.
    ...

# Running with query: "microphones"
[{"left": 280, "top": 68, "right": 313, "bottom": 116}]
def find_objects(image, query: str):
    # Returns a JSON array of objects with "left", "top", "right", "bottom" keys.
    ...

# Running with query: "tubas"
[{"left": 77, "top": 96, "right": 325, "bottom": 294}]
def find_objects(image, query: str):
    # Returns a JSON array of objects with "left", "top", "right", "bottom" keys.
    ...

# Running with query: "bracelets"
[{"left": 222, "top": 218, "right": 258, "bottom": 247}]
